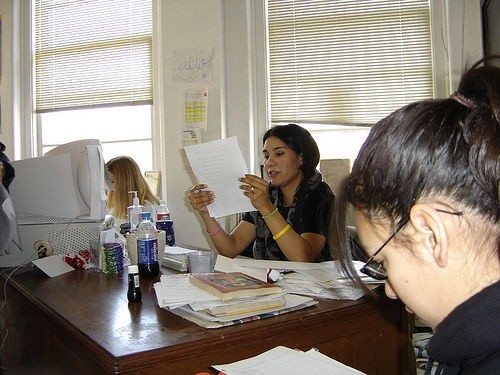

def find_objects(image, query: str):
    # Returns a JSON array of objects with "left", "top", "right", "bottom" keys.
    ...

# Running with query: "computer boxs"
[{"left": 0, "top": 214, "right": 103, "bottom": 271}]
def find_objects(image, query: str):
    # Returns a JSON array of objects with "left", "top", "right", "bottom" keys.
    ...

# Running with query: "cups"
[
  {"left": 89, "top": 239, "right": 101, "bottom": 273},
  {"left": 125, "top": 230, "right": 166, "bottom": 272},
  {"left": 102, "top": 243, "right": 124, "bottom": 274},
  {"left": 186, "top": 251, "right": 215, "bottom": 273},
  {"left": 156, "top": 219, "right": 175, "bottom": 246}
]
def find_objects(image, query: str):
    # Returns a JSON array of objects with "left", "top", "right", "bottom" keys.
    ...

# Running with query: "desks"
[{"left": 0, "top": 242, "right": 416, "bottom": 375}]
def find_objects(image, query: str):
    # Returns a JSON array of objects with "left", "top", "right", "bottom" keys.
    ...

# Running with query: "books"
[{"left": 188, "top": 271, "right": 285, "bottom": 316}]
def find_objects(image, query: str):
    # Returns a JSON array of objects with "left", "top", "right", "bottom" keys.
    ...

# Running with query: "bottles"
[
  {"left": 120, "top": 222, "right": 131, "bottom": 238},
  {"left": 127, "top": 265, "right": 142, "bottom": 303},
  {"left": 156, "top": 200, "right": 170, "bottom": 220},
  {"left": 136, "top": 211, "right": 159, "bottom": 279}
]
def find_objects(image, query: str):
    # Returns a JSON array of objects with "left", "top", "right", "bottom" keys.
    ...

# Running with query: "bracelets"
[
  {"left": 272, "top": 224, "right": 291, "bottom": 240},
  {"left": 262, "top": 208, "right": 278, "bottom": 219},
  {"left": 207, "top": 225, "right": 222, "bottom": 237}
]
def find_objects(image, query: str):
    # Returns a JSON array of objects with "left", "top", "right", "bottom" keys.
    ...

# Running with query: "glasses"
[{"left": 360, "top": 209, "right": 465, "bottom": 280}]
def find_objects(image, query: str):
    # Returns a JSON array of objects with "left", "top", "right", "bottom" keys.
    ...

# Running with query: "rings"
[
  {"left": 193, "top": 198, "right": 199, "bottom": 205},
  {"left": 251, "top": 185, "right": 255, "bottom": 194}
]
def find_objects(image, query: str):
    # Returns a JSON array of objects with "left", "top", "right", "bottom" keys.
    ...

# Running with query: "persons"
[
  {"left": 328, "top": 55, "right": 500, "bottom": 375},
  {"left": 104, "top": 156, "right": 160, "bottom": 228},
  {"left": 189, "top": 124, "right": 336, "bottom": 263}
]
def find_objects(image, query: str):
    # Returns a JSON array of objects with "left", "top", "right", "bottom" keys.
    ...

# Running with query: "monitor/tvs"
[{"left": 0, "top": 139, "right": 105, "bottom": 224}]
